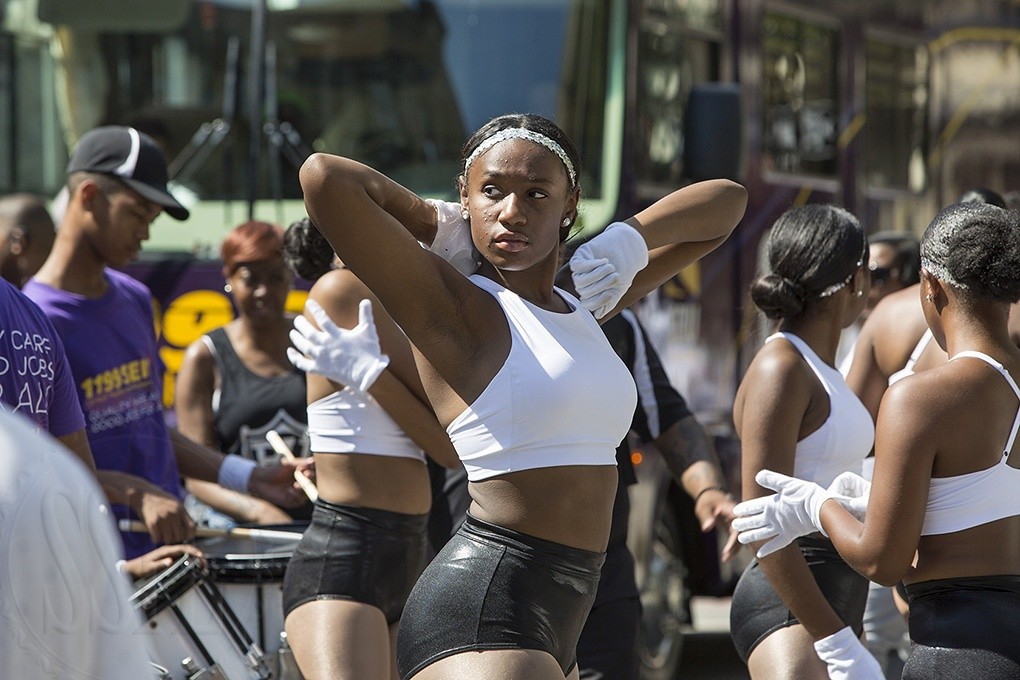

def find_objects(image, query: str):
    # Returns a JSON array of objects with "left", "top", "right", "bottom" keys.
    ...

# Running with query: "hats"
[{"left": 65, "top": 124, "right": 190, "bottom": 221}]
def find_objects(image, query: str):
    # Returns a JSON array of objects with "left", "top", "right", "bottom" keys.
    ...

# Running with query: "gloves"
[
  {"left": 862, "top": 580, "right": 912, "bottom": 663},
  {"left": 825, "top": 470, "right": 872, "bottom": 523},
  {"left": 569, "top": 221, "right": 649, "bottom": 319},
  {"left": 814, "top": 626, "right": 887, "bottom": 680},
  {"left": 731, "top": 469, "right": 834, "bottom": 558},
  {"left": 286, "top": 297, "right": 391, "bottom": 391},
  {"left": 416, "top": 197, "right": 479, "bottom": 278}
]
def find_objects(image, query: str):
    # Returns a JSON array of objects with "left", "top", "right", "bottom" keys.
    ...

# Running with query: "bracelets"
[{"left": 215, "top": 452, "right": 256, "bottom": 496}]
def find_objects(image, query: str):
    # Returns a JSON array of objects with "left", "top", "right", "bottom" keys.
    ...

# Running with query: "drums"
[
  {"left": 126, "top": 549, "right": 276, "bottom": 680},
  {"left": 193, "top": 524, "right": 303, "bottom": 679}
]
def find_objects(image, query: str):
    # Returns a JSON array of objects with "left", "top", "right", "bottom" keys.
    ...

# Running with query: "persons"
[
  {"left": 0, "top": 196, "right": 56, "bottom": 286},
  {"left": 573, "top": 310, "right": 744, "bottom": 680},
  {"left": 0, "top": 276, "right": 96, "bottom": 480},
  {"left": 292, "top": 109, "right": 748, "bottom": 678},
  {"left": 25, "top": 126, "right": 322, "bottom": 546},
  {"left": 845, "top": 279, "right": 1020, "bottom": 427},
  {"left": 846, "top": 228, "right": 930, "bottom": 377},
  {"left": 0, "top": 401, "right": 160, "bottom": 677},
  {"left": 726, "top": 190, "right": 1020, "bottom": 680},
  {"left": 174, "top": 221, "right": 322, "bottom": 524},
  {"left": 723, "top": 201, "right": 897, "bottom": 680},
  {"left": 282, "top": 214, "right": 461, "bottom": 680}
]
